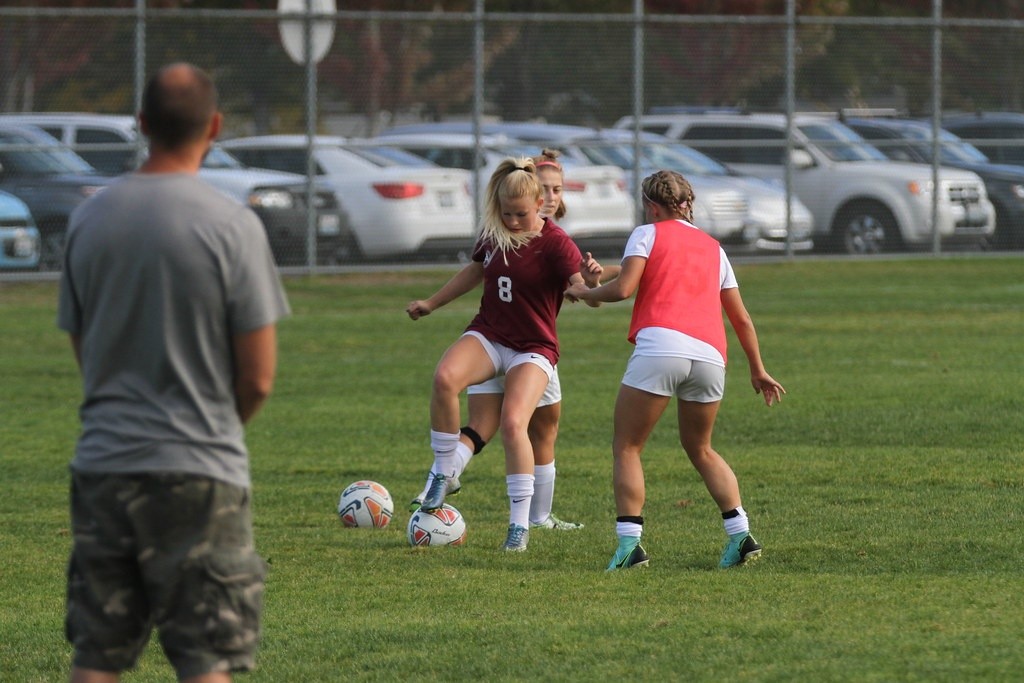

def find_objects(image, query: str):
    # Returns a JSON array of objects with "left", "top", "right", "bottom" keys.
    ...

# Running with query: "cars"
[
  {"left": 0, "top": 191, "right": 43, "bottom": 271},
  {"left": 217, "top": 134, "right": 475, "bottom": 266},
  {"left": 1, "top": 122, "right": 108, "bottom": 274},
  {"left": 381, "top": 123, "right": 816, "bottom": 257},
  {"left": 920, "top": 113, "right": 1024, "bottom": 167},
  {"left": 370, "top": 129, "right": 636, "bottom": 253}
]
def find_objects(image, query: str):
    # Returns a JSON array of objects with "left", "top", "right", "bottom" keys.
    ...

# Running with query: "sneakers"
[
  {"left": 530, "top": 513, "right": 585, "bottom": 530},
  {"left": 718, "top": 532, "right": 762, "bottom": 571},
  {"left": 605, "top": 541, "right": 649, "bottom": 573},
  {"left": 412, "top": 493, "right": 426, "bottom": 506},
  {"left": 504, "top": 523, "right": 530, "bottom": 554},
  {"left": 421, "top": 469, "right": 461, "bottom": 514}
]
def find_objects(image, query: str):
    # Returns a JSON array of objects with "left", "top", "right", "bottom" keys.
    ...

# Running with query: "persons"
[
  {"left": 563, "top": 170, "right": 786, "bottom": 576},
  {"left": 406, "top": 149, "right": 622, "bottom": 553},
  {"left": 55, "top": 63, "right": 293, "bottom": 683}
]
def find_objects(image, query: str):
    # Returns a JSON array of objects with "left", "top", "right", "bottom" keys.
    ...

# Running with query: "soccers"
[
  {"left": 338, "top": 480, "right": 394, "bottom": 528},
  {"left": 408, "top": 502, "right": 466, "bottom": 547}
]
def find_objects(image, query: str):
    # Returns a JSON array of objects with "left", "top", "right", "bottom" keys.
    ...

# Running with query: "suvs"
[
  {"left": 835, "top": 107, "right": 1024, "bottom": 251},
  {"left": 0, "top": 112, "right": 349, "bottom": 265},
  {"left": 612, "top": 104, "right": 997, "bottom": 255}
]
